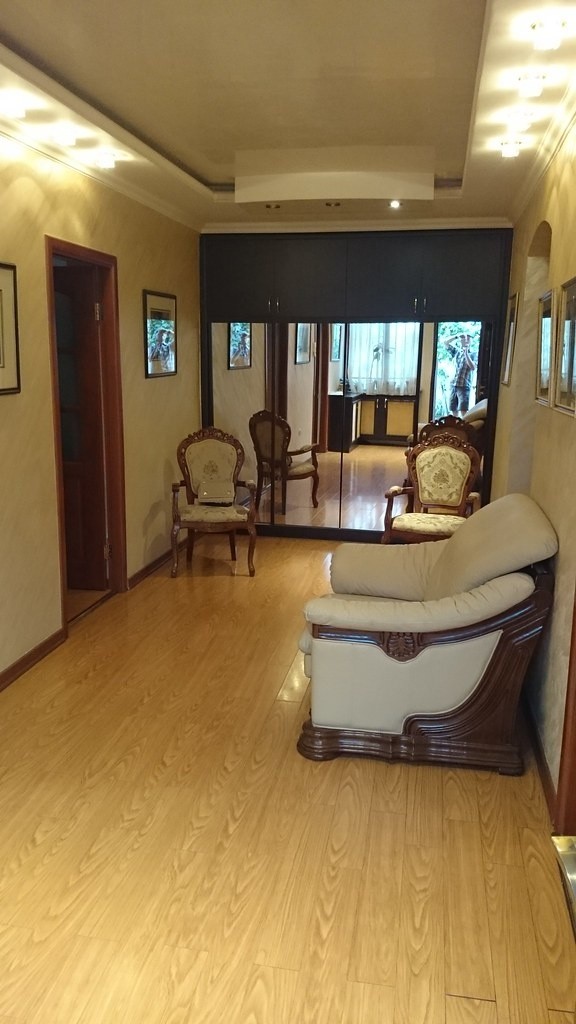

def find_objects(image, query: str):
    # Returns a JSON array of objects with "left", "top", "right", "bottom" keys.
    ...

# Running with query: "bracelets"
[{"left": 454, "top": 335, "right": 458, "bottom": 339}]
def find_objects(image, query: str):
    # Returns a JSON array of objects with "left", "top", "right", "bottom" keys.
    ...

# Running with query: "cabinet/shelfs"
[
  {"left": 362, "top": 395, "right": 417, "bottom": 446},
  {"left": 200, "top": 228, "right": 513, "bottom": 321},
  {"left": 347, "top": 392, "right": 366, "bottom": 452}
]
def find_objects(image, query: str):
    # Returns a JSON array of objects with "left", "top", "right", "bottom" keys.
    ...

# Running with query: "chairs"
[
  {"left": 403, "top": 415, "right": 478, "bottom": 516},
  {"left": 381, "top": 431, "right": 481, "bottom": 546},
  {"left": 171, "top": 425, "right": 258, "bottom": 578},
  {"left": 248, "top": 409, "right": 322, "bottom": 514}
]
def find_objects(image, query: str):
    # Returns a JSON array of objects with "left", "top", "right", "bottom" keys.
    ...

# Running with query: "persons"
[
  {"left": 231, "top": 332, "right": 250, "bottom": 367},
  {"left": 444, "top": 333, "right": 475, "bottom": 420},
  {"left": 149, "top": 328, "right": 175, "bottom": 373}
]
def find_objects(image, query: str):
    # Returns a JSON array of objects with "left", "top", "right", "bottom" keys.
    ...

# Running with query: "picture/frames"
[
  {"left": 534, "top": 287, "right": 558, "bottom": 410},
  {"left": 330, "top": 323, "right": 342, "bottom": 361},
  {"left": 294, "top": 323, "right": 310, "bottom": 365},
  {"left": 227, "top": 323, "right": 252, "bottom": 370},
  {"left": 554, "top": 276, "right": 576, "bottom": 418},
  {"left": 0, "top": 261, "right": 22, "bottom": 395},
  {"left": 501, "top": 291, "right": 519, "bottom": 386},
  {"left": 142, "top": 288, "right": 178, "bottom": 379}
]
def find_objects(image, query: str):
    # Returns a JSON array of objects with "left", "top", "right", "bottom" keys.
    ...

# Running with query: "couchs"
[
  {"left": 408, "top": 399, "right": 489, "bottom": 452},
  {"left": 296, "top": 493, "right": 554, "bottom": 777}
]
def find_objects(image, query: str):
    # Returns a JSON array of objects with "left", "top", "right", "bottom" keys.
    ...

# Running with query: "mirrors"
[{"left": 201, "top": 320, "right": 503, "bottom": 543}]
